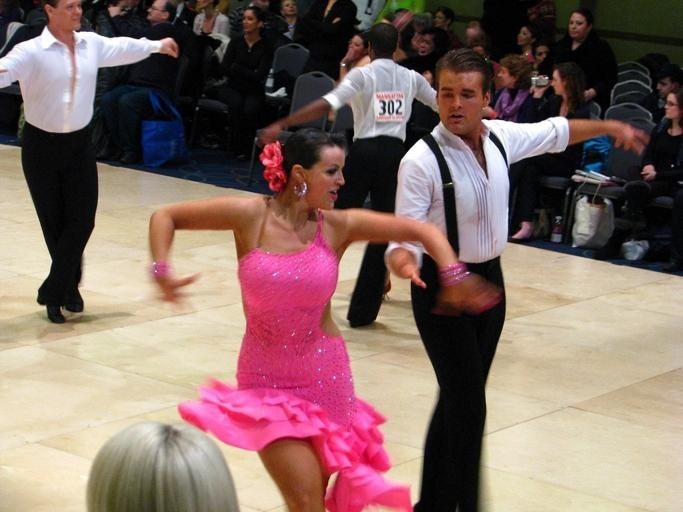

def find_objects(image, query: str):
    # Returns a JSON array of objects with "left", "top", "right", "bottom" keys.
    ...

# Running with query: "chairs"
[
  {"left": 199, "top": 43, "right": 354, "bottom": 185},
  {"left": 509, "top": 61, "right": 656, "bottom": 243}
]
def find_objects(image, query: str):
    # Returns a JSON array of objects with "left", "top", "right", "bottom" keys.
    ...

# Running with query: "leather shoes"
[
  {"left": 66, "top": 287, "right": 84, "bottom": 312},
  {"left": 38, "top": 293, "right": 66, "bottom": 323}
]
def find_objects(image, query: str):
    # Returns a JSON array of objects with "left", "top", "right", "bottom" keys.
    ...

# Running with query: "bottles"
[
  {"left": 550, "top": 215, "right": 562, "bottom": 243},
  {"left": 265, "top": 66, "right": 274, "bottom": 95}
]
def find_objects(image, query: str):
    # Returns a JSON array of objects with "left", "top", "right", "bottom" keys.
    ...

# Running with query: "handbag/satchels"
[
  {"left": 140, "top": 88, "right": 187, "bottom": 172},
  {"left": 571, "top": 196, "right": 615, "bottom": 249}
]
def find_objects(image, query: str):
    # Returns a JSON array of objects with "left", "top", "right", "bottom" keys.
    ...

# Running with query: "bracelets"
[
  {"left": 438, "top": 261, "right": 471, "bottom": 287},
  {"left": 148, "top": 258, "right": 172, "bottom": 281}
]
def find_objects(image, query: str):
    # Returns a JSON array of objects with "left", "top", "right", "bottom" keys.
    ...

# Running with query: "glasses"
[{"left": 666, "top": 100, "right": 677, "bottom": 107}]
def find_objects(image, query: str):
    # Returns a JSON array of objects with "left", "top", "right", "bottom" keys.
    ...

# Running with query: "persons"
[
  {"left": 1, "top": 1, "right": 683, "bottom": 272},
  {"left": 382, "top": 45, "right": 649, "bottom": 512},
  {"left": 0, "top": 0, "right": 179, "bottom": 325},
  {"left": 84, "top": 419, "right": 240, "bottom": 512},
  {"left": 147, "top": 126, "right": 506, "bottom": 512},
  {"left": 254, "top": 21, "right": 441, "bottom": 330}
]
